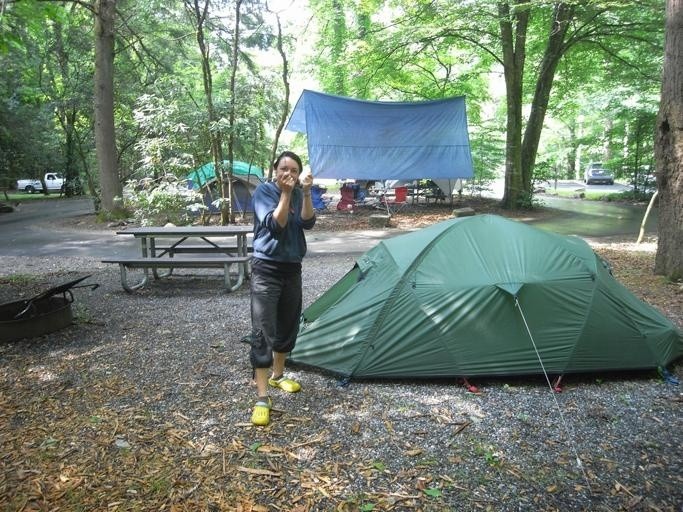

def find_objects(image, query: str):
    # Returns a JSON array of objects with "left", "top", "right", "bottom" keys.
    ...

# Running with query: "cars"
[
  {"left": 637, "top": 165, "right": 657, "bottom": 186},
  {"left": 583, "top": 162, "right": 614, "bottom": 185}
]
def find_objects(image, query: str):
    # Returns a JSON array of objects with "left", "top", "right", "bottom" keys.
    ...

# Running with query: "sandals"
[
  {"left": 267, "top": 374, "right": 303, "bottom": 394},
  {"left": 248, "top": 397, "right": 273, "bottom": 427}
]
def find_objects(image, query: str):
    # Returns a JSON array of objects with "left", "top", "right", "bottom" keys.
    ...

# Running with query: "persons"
[{"left": 247, "top": 149, "right": 318, "bottom": 427}]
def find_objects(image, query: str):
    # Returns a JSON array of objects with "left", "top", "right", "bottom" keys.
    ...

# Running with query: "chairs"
[{"left": 312, "top": 186, "right": 444, "bottom": 211}]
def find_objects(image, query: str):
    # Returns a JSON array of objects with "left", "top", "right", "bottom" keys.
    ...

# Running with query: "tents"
[
  {"left": 173, "top": 158, "right": 266, "bottom": 215},
  {"left": 237, "top": 211, "right": 682, "bottom": 397}
]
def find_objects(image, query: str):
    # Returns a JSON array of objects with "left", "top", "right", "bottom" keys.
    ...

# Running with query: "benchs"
[
  {"left": 102, "top": 257, "right": 250, "bottom": 293},
  {"left": 148, "top": 245, "right": 254, "bottom": 276}
]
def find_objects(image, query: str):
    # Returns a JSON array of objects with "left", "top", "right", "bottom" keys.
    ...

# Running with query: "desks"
[{"left": 115, "top": 227, "right": 255, "bottom": 282}]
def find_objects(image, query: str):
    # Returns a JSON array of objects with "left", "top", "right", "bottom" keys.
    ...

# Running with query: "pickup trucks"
[{"left": 16, "top": 172, "right": 66, "bottom": 194}]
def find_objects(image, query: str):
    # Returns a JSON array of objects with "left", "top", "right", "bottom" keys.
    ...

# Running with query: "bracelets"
[{"left": 302, "top": 192, "right": 310, "bottom": 198}]
